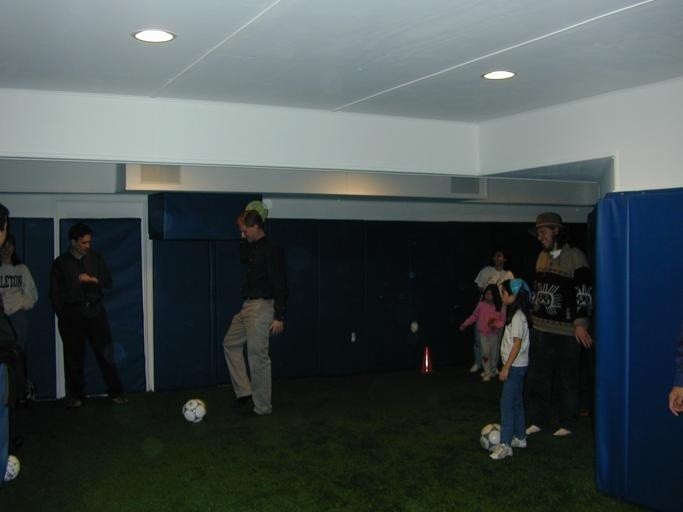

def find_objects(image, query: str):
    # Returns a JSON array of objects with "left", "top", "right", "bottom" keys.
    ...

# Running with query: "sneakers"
[
  {"left": 470, "top": 363, "right": 499, "bottom": 381},
  {"left": 229, "top": 395, "right": 272, "bottom": 417},
  {"left": 511, "top": 437, "right": 527, "bottom": 448},
  {"left": 67, "top": 399, "right": 86, "bottom": 408},
  {"left": 489, "top": 443, "right": 513, "bottom": 460},
  {"left": 109, "top": 395, "right": 129, "bottom": 405}
]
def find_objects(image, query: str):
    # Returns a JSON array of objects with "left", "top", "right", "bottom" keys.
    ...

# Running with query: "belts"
[
  {"left": 66, "top": 298, "right": 101, "bottom": 306},
  {"left": 243, "top": 294, "right": 274, "bottom": 301}
]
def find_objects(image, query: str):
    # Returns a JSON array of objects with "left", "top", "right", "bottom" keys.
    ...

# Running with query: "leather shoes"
[{"left": 9, "top": 434, "right": 24, "bottom": 455}]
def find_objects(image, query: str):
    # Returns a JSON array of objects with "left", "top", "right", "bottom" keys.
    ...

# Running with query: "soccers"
[
  {"left": 3, "top": 455, "right": 20, "bottom": 481},
  {"left": 480, "top": 424, "right": 501, "bottom": 450},
  {"left": 182, "top": 399, "right": 206, "bottom": 423}
]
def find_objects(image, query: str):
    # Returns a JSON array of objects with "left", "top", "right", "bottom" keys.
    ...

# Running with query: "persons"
[
  {"left": 0, "top": 232, "right": 40, "bottom": 410},
  {"left": 469, "top": 248, "right": 517, "bottom": 379},
  {"left": 667, "top": 339, "right": 682, "bottom": 419},
  {"left": 486, "top": 277, "right": 532, "bottom": 462},
  {"left": 46, "top": 221, "right": 132, "bottom": 409},
  {"left": 456, "top": 283, "right": 507, "bottom": 384},
  {"left": 524, "top": 211, "right": 594, "bottom": 436},
  {"left": 220, "top": 209, "right": 287, "bottom": 417},
  {"left": 0, "top": 202, "right": 15, "bottom": 501}
]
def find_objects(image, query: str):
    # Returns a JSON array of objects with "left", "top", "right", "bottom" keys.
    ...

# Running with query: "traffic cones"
[{"left": 415, "top": 343, "right": 433, "bottom": 378}]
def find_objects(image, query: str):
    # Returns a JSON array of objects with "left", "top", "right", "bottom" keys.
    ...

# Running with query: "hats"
[{"left": 528, "top": 212, "right": 566, "bottom": 238}]
